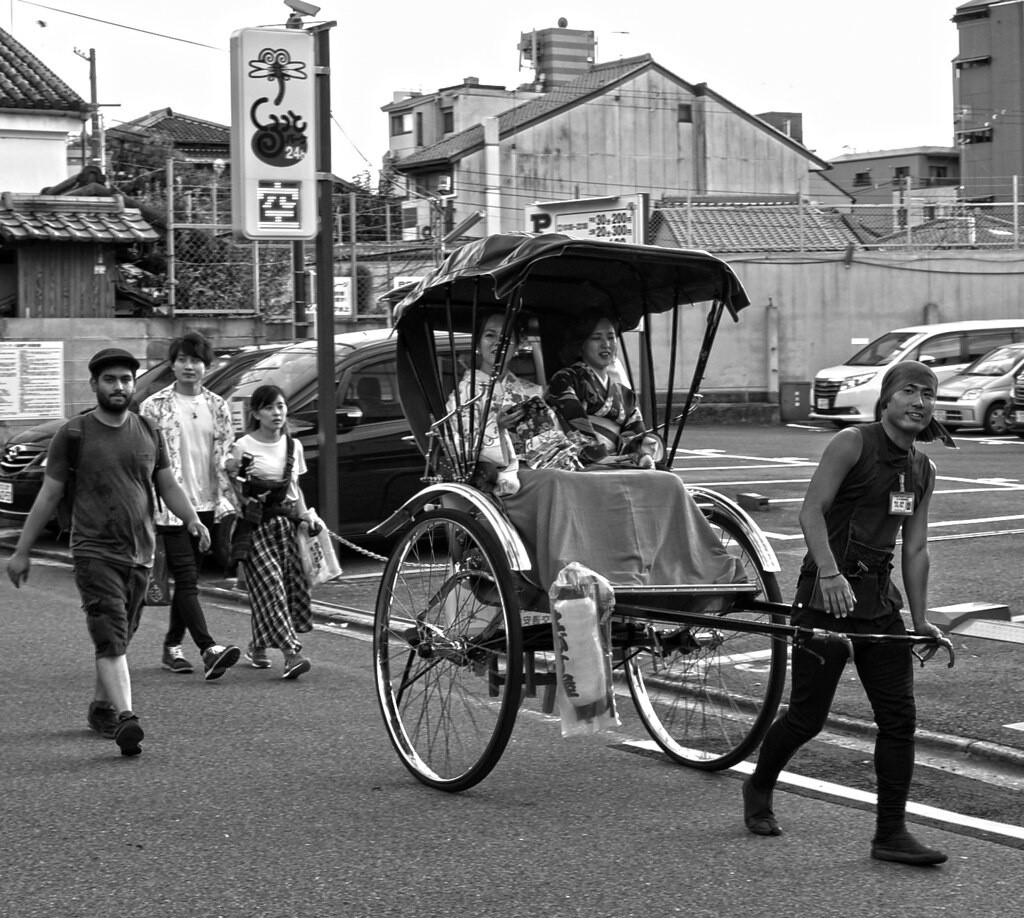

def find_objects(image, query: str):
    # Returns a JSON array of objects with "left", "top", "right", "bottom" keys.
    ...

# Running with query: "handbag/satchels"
[
  {"left": 297, "top": 516, "right": 344, "bottom": 598},
  {"left": 240, "top": 472, "right": 291, "bottom": 525}
]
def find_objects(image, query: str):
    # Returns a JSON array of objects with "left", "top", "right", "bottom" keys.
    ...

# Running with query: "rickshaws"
[{"left": 367, "top": 232, "right": 956, "bottom": 798}]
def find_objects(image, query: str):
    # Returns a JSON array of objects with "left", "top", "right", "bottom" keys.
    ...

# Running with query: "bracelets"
[{"left": 818, "top": 573, "right": 842, "bottom": 579}]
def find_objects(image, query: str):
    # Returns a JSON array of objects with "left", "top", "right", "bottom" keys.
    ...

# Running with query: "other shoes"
[
  {"left": 870, "top": 833, "right": 949, "bottom": 866},
  {"left": 114, "top": 716, "right": 144, "bottom": 756},
  {"left": 87, "top": 700, "right": 119, "bottom": 738},
  {"left": 742, "top": 774, "right": 783, "bottom": 836}
]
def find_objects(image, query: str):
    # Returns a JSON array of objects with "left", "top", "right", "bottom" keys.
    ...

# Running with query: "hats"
[{"left": 88, "top": 347, "right": 140, "bottom": 375}]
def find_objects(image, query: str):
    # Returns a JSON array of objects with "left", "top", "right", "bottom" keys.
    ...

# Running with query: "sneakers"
[
  {"left": 281, "top": 653, "right": 312, "bottom": 680},
  {"left": 160, "top": 641, "right": 194, "bottom": 673},
  {"left": 201, "top": 643, "right": 241, "bottom": 680},
  {"left": 244, "top": 641, "right": 272, "bottom": 668}
]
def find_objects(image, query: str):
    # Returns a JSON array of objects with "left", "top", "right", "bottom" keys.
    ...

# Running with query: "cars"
[
  {"left": 932, "top": 342, "right": 1024, "bottom": 437},
  {"left": 0, "top": 336, "right": 310, "bottom": 529},
  {"left": 195, "top": 329, "right": 633, "bottom": 575},
  {"left": 1001, "top": 369, "right": 1024, "bottom": 440}
]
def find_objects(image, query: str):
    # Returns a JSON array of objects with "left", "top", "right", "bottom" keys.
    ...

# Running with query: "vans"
[{"left": 807, "top": 318, "right": 1024, "bottom": 432}]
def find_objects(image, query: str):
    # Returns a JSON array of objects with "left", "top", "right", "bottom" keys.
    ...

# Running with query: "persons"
[
  {"left": 138, "top": 333, "right": 241, "bottom": 679},
  {"left": 445, "top": 315, "right": 671, "bottom": 503},
  {"left": 741, "top": 360, "right": 952, "bottom": 865},
  {"left": 233, "top": 385, "right": 319, "bottom": 678},
  {"left": 7, "top": 348, "right": 211, "bottom": 754}
]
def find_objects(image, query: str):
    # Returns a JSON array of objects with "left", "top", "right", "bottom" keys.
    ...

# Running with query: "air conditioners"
[{"left": 417, "top": 224, "right": 434, "bottom": 239}]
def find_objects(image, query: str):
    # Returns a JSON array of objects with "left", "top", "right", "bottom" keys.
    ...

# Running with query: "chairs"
[{"left": 357, "top": 377, "right": 388, "bottom": 418}]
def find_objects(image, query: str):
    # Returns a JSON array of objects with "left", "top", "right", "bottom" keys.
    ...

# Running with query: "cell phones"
[
  {"left": 236, "top": 452, "right": 254, "bottom": 482},
  {"left": 507, "top": 396, "right": 555, "bottom": 442}
]
